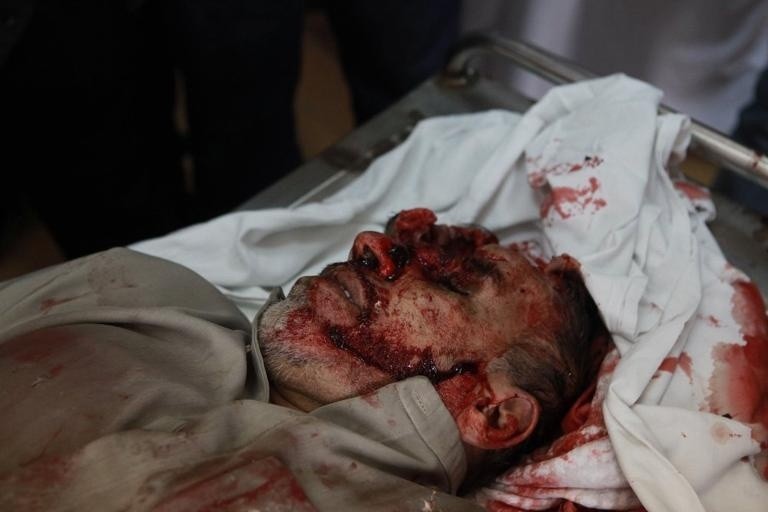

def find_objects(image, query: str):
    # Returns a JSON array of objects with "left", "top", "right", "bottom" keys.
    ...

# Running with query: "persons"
[{"left": 0, "top": 204, "right": 612, "bottom": 512}]
